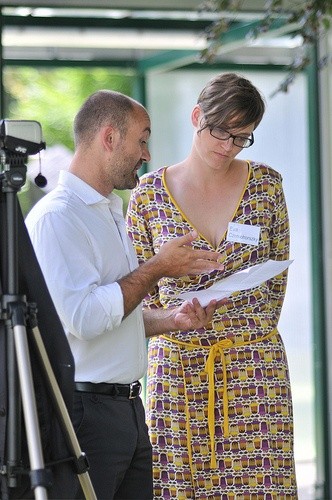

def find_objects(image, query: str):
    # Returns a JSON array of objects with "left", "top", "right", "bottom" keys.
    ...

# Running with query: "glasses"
[{"left": 205, "top": 115, "right": 254, "bottom": 148}]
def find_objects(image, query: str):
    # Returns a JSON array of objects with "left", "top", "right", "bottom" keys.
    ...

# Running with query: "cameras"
[{"left": 0, "top": 120, "right": 46, "bottom": 155}]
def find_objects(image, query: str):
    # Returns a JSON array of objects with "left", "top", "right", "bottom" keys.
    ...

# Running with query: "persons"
[
  {"left": 25, "top": 90, "right": 228, "bottom": 500},
  {"left": 125, "top": 73, "right": 298, "bottom": 500}
]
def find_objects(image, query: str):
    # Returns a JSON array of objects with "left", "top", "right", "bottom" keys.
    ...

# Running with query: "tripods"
[{"left": 0, "top": 153, "right": 98, "bottom": 500}]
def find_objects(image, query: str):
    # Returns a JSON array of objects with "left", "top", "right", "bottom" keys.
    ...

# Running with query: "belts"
[{"left": 73, "top": 381, "right": 142, "bottom": 399}]
